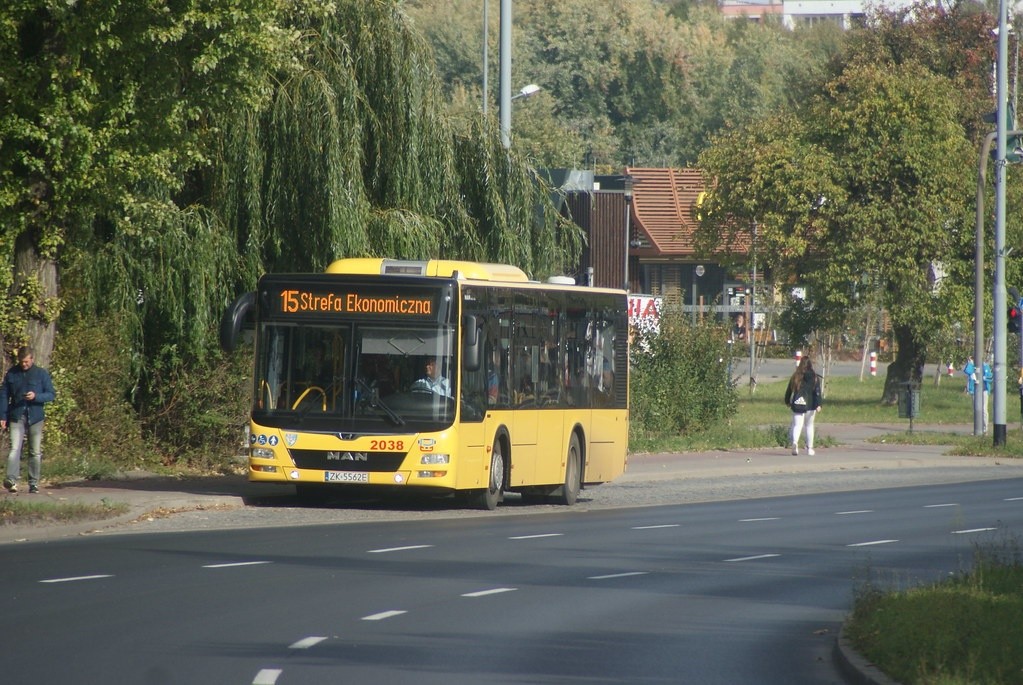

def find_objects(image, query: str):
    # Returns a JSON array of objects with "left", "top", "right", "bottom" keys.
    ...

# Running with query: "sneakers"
[
  {"left": 28, "top": 484, "right": 39, "bottom": 493},
  {"left": 3, "top": 478, "right": 18, "bottom": 493}
]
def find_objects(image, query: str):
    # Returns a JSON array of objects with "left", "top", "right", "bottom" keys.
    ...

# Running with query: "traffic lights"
[{"left": 1007, "top": 307, "right": 1020, "bottom": 333}]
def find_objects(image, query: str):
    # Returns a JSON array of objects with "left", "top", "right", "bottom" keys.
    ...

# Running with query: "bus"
[{"left": 220, "top": 258, "right": 631, "bottom": 511}]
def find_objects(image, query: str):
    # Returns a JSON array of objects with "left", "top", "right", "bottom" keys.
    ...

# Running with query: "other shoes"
[
  {"left": 791, "top": 442, "right": 798, "bottom": 455},
  {"left": 807, "top": 449, "right": 815, "bottom": 456}
]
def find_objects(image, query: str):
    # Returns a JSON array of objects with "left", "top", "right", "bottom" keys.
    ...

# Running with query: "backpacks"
[{"left": 791, "top": 382, "right": 813, "bottom": 414}]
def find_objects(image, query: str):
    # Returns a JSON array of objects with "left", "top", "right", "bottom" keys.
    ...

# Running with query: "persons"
[
  {"left": 0, "top": 346, "right": 56, "bottom": 496},
  {"left": 785, "top": 356, "right": 823, "bottom": 456},
  {"left": 964, "top": 354, "right": 992, "bottom": 435},
  {"left": 409, "top": 356, "right": 451, "bottom": 398},
  {"left": 1018, "top": 367, "right": 1023, "bottom": 422}
]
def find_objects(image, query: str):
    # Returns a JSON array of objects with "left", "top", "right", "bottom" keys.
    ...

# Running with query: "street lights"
[
  {"left": 499, "top": 84, "right": 543, "bottom": 153},
  {"left": 616, "top": 174, "right": 641, "bottom": 291}
]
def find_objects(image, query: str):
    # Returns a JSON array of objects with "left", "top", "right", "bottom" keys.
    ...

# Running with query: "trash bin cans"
[{"left": 895, "top": 382, "right": 920, "bottom": 418}]
